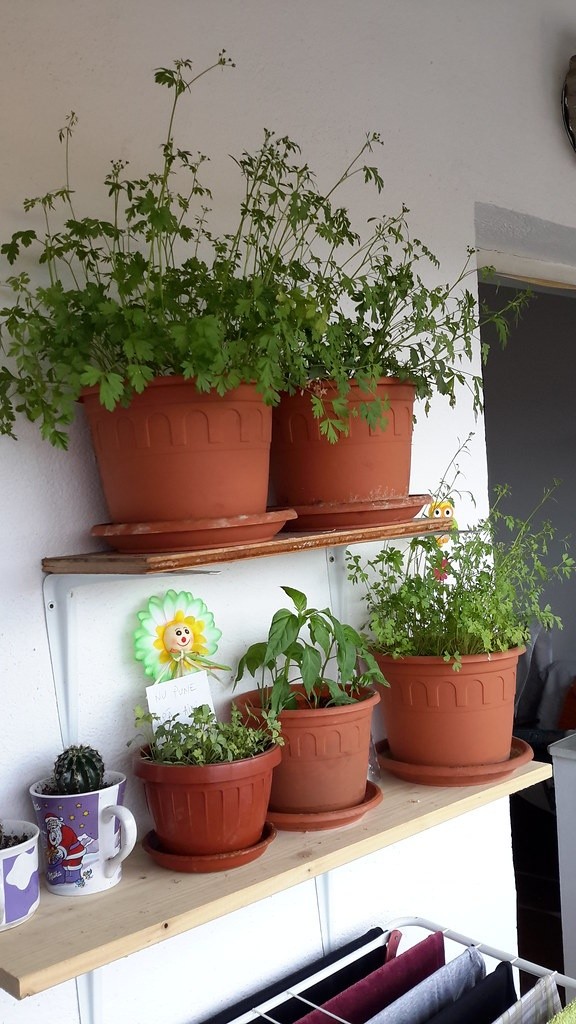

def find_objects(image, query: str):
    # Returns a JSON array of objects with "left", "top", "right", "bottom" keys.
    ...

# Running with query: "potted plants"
[
  {"left": 275, "top": 183, "right": 549, "bottom": 537},
  {"left": 359, "top": 436, "right": 576, "bottom": 783},
  {"left": 119, "top": 700, "right": 286, "bottom": 873},
  {"left": 24, "top": 740, "right": 136, "bottom": 899},
  {"left": 0, "top": 48, "right": 384, "bottom": 549},
  {"left": 227, "top": 580, "right": 396, "bottom": 833}
]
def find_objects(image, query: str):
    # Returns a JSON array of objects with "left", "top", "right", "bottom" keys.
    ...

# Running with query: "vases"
[{"left": 0, "top": 817, "right": 42, "bottom": 934}]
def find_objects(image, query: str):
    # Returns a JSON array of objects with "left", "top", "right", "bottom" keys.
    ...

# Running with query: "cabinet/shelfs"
[{"left": 0, "top": 518, "right": 554, "bottom": 1002}]
